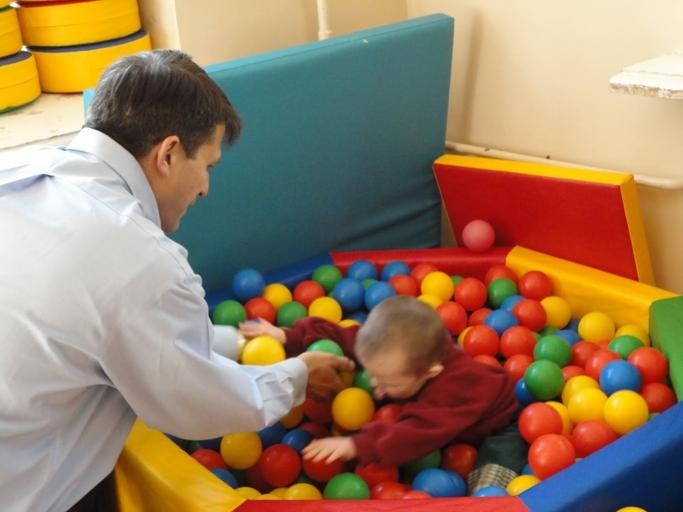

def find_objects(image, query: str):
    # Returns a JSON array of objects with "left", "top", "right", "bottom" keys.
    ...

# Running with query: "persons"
[
  {"left": 2, "top": 48, "right": 357, "bottom": 511},
  {"left": 238, "top": 295, "right": 529, "bottom": 496}
]
